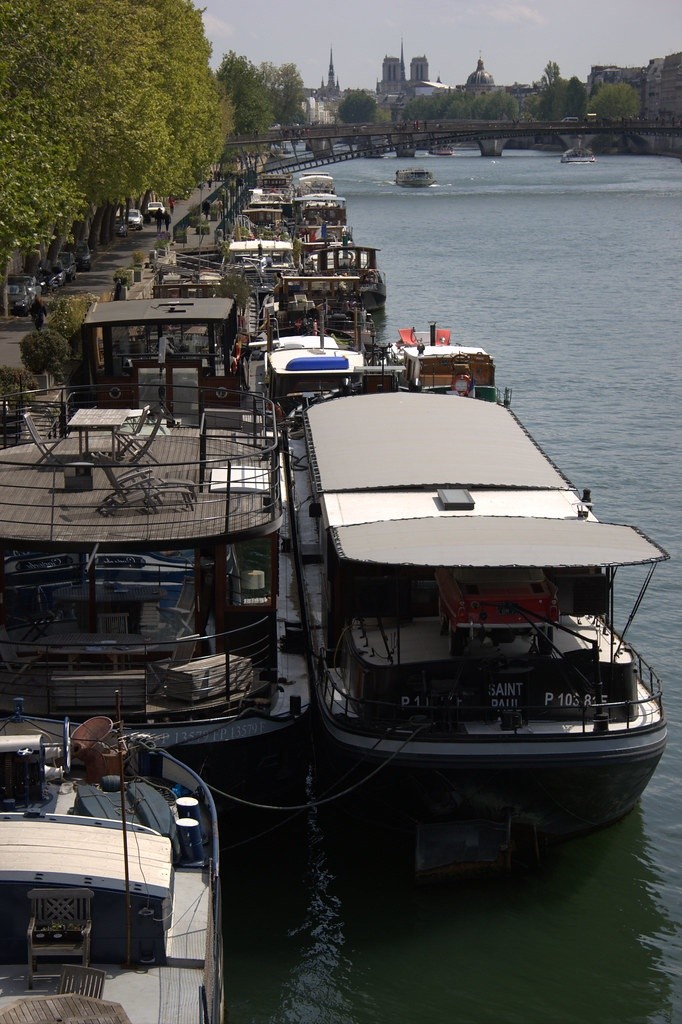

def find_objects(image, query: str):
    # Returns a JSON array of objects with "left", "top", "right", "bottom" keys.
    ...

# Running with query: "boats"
[
  {"left": 560, "top": 149, "right": 596, "bottom": 163},
  {"left": 394, "top": 167, "right": 437, "bottom": 188},
  {"left": 0, "top": 692, "right": 234, "bottom": 1024},
  {"left": 284, "top": 387, "right": 671, "bottom": 854},
  {"left": 0, "top": 170, "right": 512, "bottom": 819},
  {"left": 429, "top": 144, "right": 454, "bottom": 156}
]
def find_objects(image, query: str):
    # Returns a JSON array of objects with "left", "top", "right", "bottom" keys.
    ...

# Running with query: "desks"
[
  {"left": 51, "top": 581, "right": 167, "bottom": 632},
  {"left": 67, "top": 408, "right": 131, "bottom": 465},
  {"left": 35, "top": 632, "right": 160, "bottom": 671},
  {"left": 0, "top": 992, "right": 133, "bottom": 1024}
]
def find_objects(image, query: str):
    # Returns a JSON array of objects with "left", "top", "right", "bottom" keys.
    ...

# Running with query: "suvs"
[
  {"left": 7, "top": 272, "right": 44, "bottom": 317},
  {"left": 123, "top": 208, "right": 144, "bottom": 230},
  {"left": 58, "top": 251, "right": 79, "bottom": 283}
]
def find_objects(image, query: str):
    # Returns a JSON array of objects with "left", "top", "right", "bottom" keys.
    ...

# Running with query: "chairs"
[
  {"left": 93, "top": 405, "right": 198, "bottom": 518},
  {"left": 97, "top": 573, "right": 201, "bottom": 700},
  {"left": 0, "top": 584, "right": 65, "bottom": 688},
  {"left": 57, "top": 964, "right": 106, "bottom": 999},
  {"left": 23, "top": 412, "right": 65, "bottom": 471},
  {"left": 27, "top": 887, "right": 94, "bottom": 989}
]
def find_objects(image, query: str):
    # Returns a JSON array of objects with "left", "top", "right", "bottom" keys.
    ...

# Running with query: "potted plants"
[{"left": 32, "top": 919, "right": 84, "bottom": 944}]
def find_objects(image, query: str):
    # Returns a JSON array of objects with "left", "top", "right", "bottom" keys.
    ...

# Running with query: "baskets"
[{"left": 69, "top": 715, "right": 114, "bottom": 760}]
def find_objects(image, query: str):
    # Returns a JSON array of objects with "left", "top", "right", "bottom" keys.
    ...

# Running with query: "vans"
[{"left": 561, "top": 116, "right": 579, "bottom": 123}]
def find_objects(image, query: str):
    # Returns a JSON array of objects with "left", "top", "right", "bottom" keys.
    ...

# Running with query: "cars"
[
  {"left": 65, "top": 243, "right": 95, "bottom": 272},
  {"left": 40, "top": 260, "right": 67, "bottom": 288},
  {"left": 147, "top": 201, "right": 166, "bottom": 217},
  {"left": 115, "top": 220, "right": 129, "bottom": 238}
]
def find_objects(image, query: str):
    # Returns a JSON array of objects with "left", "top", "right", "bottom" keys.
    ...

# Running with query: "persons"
[
  {"left": 155, "top": 208, "right": 171, "bottom": 232},
  {"left": 169, "top": 196, "right": 176, "bottom": 213},
  {"left": 218, "top": 202, "right": 223, "bottom": 219},
  {"left": 207, "top": 180, "right": 212, "bottom": 188},
  {"left": 202, "top": 200, "right": 210, "bottom": 219},
  {"left": 214, "top": 171, "right": 220, "bottom": 181},
  {"left": 29, "top": 295, "right": 47, "bottom": 330}
]
